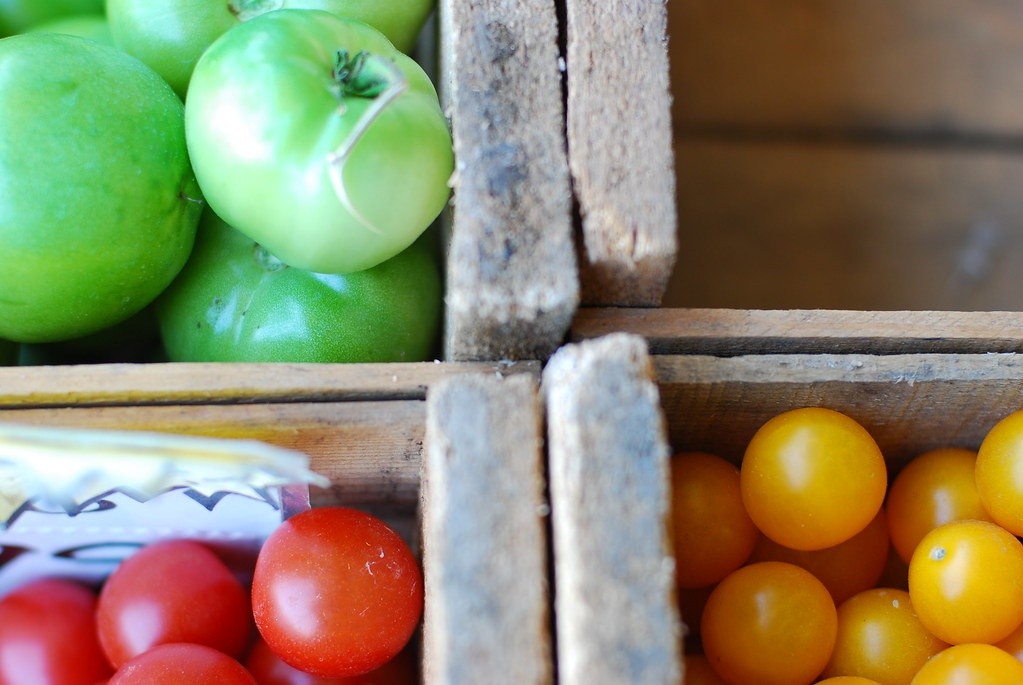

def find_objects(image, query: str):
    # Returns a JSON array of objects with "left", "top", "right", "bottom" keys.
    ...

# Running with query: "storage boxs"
[{"left": 0, "top": 0, "right": 1023, "bottom": 685}]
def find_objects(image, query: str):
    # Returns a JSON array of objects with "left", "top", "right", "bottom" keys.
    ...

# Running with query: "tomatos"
[
  {"left": 0, "top": 0, "right": 454, "bottom": 367},
  {"left": 0, "top": 506, "right": 423, "bottom": 685},
  {"left": 669, "top": 408, "right": 1023, "bottom": 685}
]
